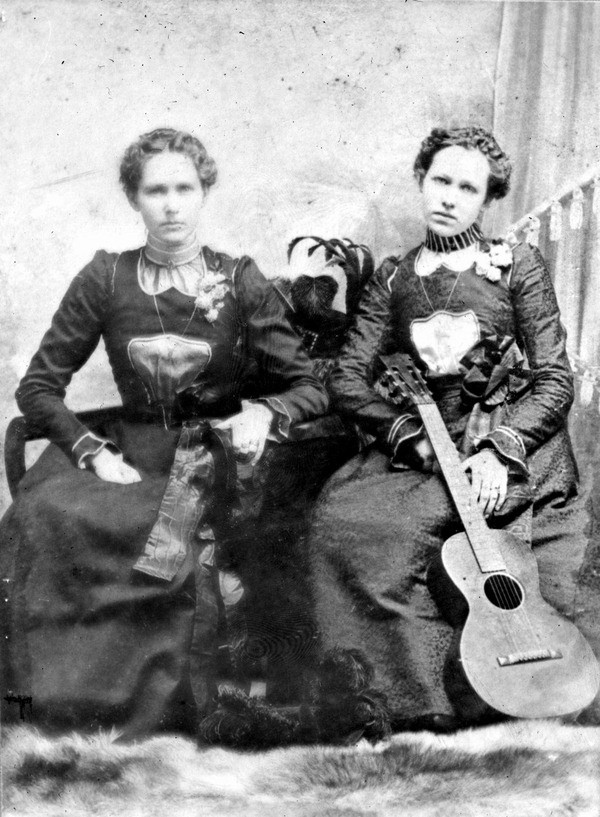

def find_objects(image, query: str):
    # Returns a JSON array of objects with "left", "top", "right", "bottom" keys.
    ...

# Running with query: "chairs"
[{"left": 4, "top": 304, "right": 349, "bottom": 708}]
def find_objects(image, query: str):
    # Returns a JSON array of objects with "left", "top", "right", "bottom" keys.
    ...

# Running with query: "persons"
[
  {"left": 244, "top": 125, "right": 600, "bottom": 733},
  {"left": 0, "top": 127, "right": 330, "bottom": 746}
]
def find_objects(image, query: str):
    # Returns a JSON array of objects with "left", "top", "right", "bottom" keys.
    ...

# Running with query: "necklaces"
[
  {"left": 152, "top": 288, "right": 198, "bottom": 357},
  {"left": 417, "top": 269, "right": 462, "bottom": 325}
]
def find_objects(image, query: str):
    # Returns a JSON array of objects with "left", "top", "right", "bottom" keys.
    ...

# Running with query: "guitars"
[{"left": 378, "top": 352, "right": 600, "bottom": 720}]
